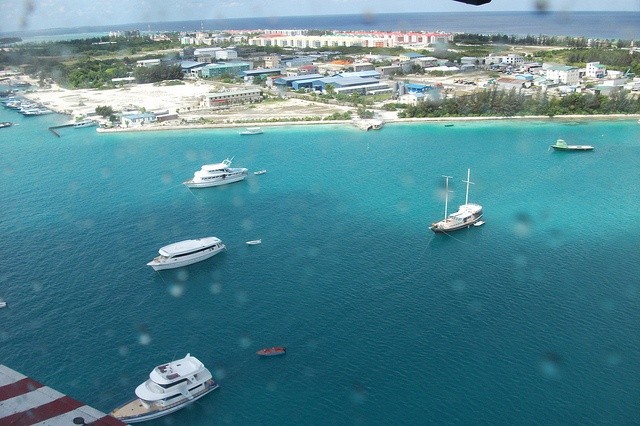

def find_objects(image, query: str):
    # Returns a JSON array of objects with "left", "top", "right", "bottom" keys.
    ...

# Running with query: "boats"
[
  {"left": 0, "top": 122, "right": 12, "bottom": 128},
  {"left": 254, "top": 169, "right": 266, "bottom": 174},
  {"left": 6, "top": 100, "right": 52, "bottom": 117},
  {"left": 256, "top": 347, "right": 285, "bottom": 356},
  {"left": 245, "top": 239, "right": 261, "bottom": 244},
  {"left": 73, "top": 120, "right": 93, "bottom": 127},
  {"left": 550, "top": 139, "right": 594, "bottom": 150},
  {"left": 372, "top": 121, "right": 383, "bottom": 129}
]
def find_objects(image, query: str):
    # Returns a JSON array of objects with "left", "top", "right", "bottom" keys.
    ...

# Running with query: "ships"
[
  {"left": 239, "top": 126, "right": 263, "bottom": 134},
  {"left": 146, "top": 237, "right": 226, "bottom": 271},
  {"left": 110, "top": 352, "right": 219, "bottom": 423},
  {"left": 182, "top": 157, "right": 248, "bottom": 188}
]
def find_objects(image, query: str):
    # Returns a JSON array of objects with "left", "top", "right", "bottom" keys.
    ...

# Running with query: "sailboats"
[{"left": 428, "top": 168, "right": 485, "bottom": 231}]
